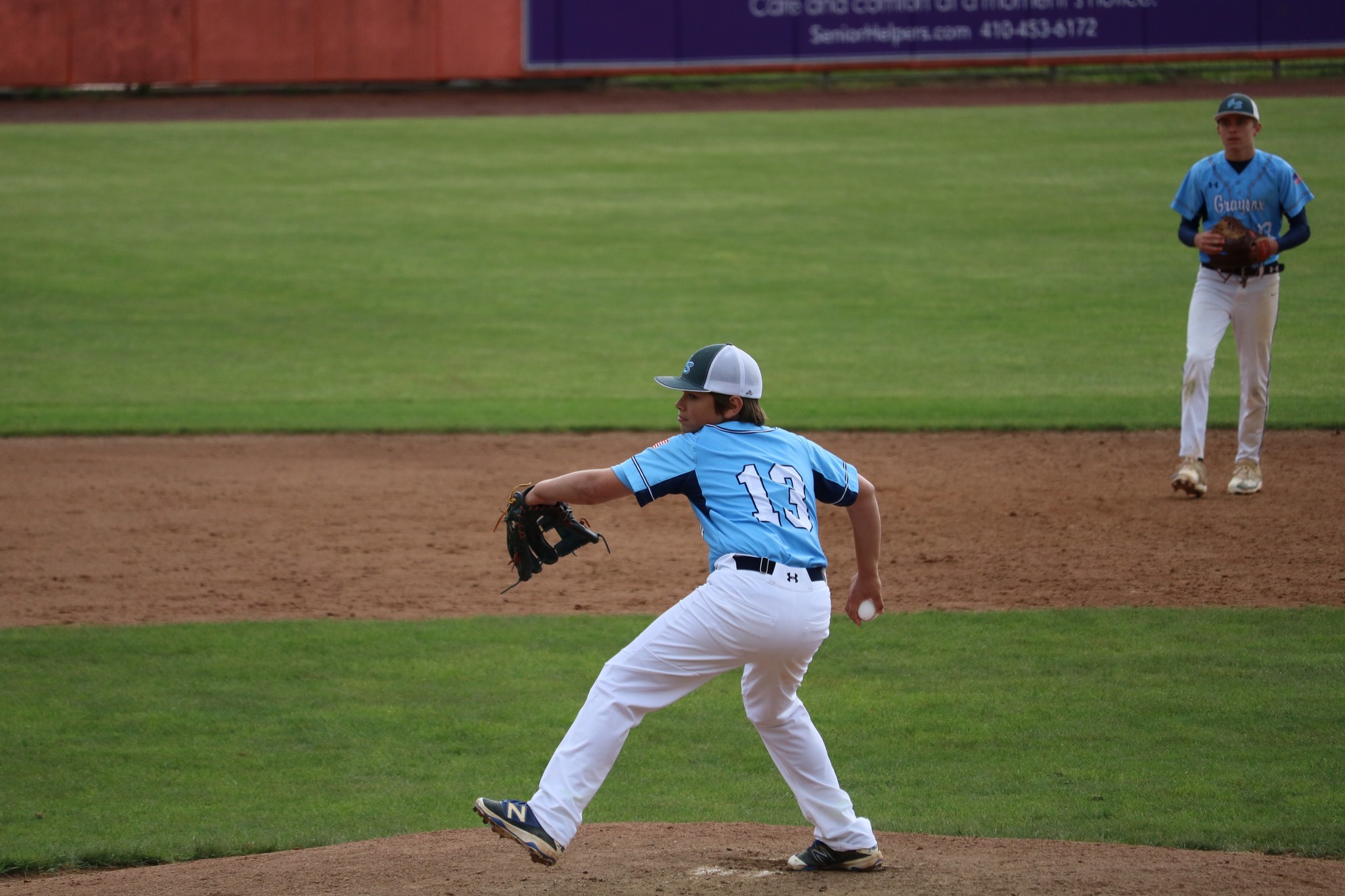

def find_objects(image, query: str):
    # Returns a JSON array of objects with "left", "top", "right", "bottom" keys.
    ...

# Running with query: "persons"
[
  {"left": 470, "top": 343, "right": 885, "bottom": 872},
  {"left": 1169, "top": 92, "right": 1315, "bottom": 499}
]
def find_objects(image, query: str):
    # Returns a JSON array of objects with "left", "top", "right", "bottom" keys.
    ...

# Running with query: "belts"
[
  {"left": 1202, "top": 260, "right": 1286, "bottom": 278},
  {"left": 730, "top": 553, "right": 826, "bottom": 583}
]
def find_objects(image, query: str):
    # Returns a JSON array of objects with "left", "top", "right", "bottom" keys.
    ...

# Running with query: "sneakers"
[
  {"left": 1228, "top": 458, "right": 1263, "bottom": 495},
  {"left": 474, "top": 797, "right": 565, "bottom": 868},
  {"left": 787, "top": 836, "right": 885, "bottom": 871},
  {"left": 1170, "top": 458, "right": 1209, "bottom": 497}
]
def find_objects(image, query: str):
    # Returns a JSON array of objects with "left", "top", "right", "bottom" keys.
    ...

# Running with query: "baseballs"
[{"left": 858, "top": 598, "right": 880, "bottom": 621}]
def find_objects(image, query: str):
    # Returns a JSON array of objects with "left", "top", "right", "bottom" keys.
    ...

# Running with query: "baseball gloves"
[
  {"left": 505, "top": 486, "right": 599, "bottom": 581},
  {"left": 1201, "top": 215, "right": 1270, "bottom": 276}
]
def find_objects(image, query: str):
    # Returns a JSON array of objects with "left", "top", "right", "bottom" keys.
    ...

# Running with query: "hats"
[
  {"left": 1215, "top": 93, "right": 1260, "bottom": 124},
  {"left": 653, "top": 342, "right": 762, "bottom": 400}
]
